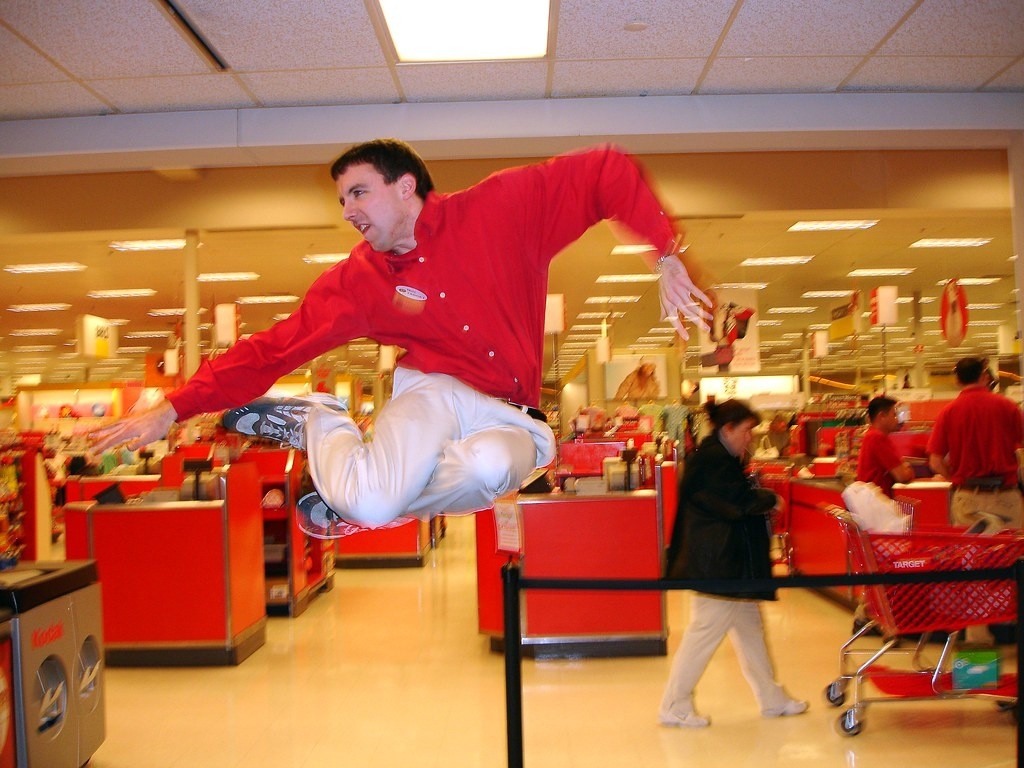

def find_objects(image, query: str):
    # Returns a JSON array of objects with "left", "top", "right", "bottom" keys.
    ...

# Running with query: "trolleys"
[{"left": 824, "top": 504, "right": 1024, "bottom": 737}]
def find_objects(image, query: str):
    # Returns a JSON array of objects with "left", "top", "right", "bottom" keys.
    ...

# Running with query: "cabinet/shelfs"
[
  {"left": 558, "top": 415, "right": 681, "bottom": 548},
  {"left": 474, "top": 460, "right": 669, "bottom": 660},
  {"left": 788, "top": 394, "right": 1024, "bottom": 619},
  {"left": 1, "top": 428, "right": 449, "bottom": 619},
  {"left": 63, "top": 462, "right": 270, "bottom": 667}
]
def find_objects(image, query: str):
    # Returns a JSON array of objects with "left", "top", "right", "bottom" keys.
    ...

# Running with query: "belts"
[
  {"left": 954, "top": 486, "right": 1014, "bottom": 493},
  {"left": 496, "top": 398, "right": 549, "bottom": 423}
]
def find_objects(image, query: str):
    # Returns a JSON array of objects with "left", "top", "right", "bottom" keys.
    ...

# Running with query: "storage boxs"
[{"left": 950, "top": 648, "right": 1000, "bottom": 688}]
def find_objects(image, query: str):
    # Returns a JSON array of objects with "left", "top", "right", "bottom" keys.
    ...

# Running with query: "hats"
[{"left": 640, "top": 355, "right": 657, "bottom": 367}]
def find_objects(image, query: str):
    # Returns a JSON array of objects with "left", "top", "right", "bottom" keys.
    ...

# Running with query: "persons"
[
  {"left": 923, "top": 357, "right": 1024, "bottom": 660},
  {"left": 614, "top": 355, "right": 662, "bottom": 398},
  {"left": 654, "top": 394, "right": 811, "bottom": 733},
  {"left": 82, "top": 134, "right": 717, "bottom": 542},
  {"left": 850, "top": 395, "right": 916, "bottom": 640}
]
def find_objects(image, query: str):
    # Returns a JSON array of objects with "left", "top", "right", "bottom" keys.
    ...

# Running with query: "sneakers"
[
  {"left": 222, "top": 391, "right": 349, "bottom": 452},
  {"left": 762, "top": 698, "right": 808, "bottom": 716},
  {"left": 658, "top": 710, "right": 711, "bottom": 727},
  {"left": 295, "top": 490, "right": 413, "bottom": 539}
]
